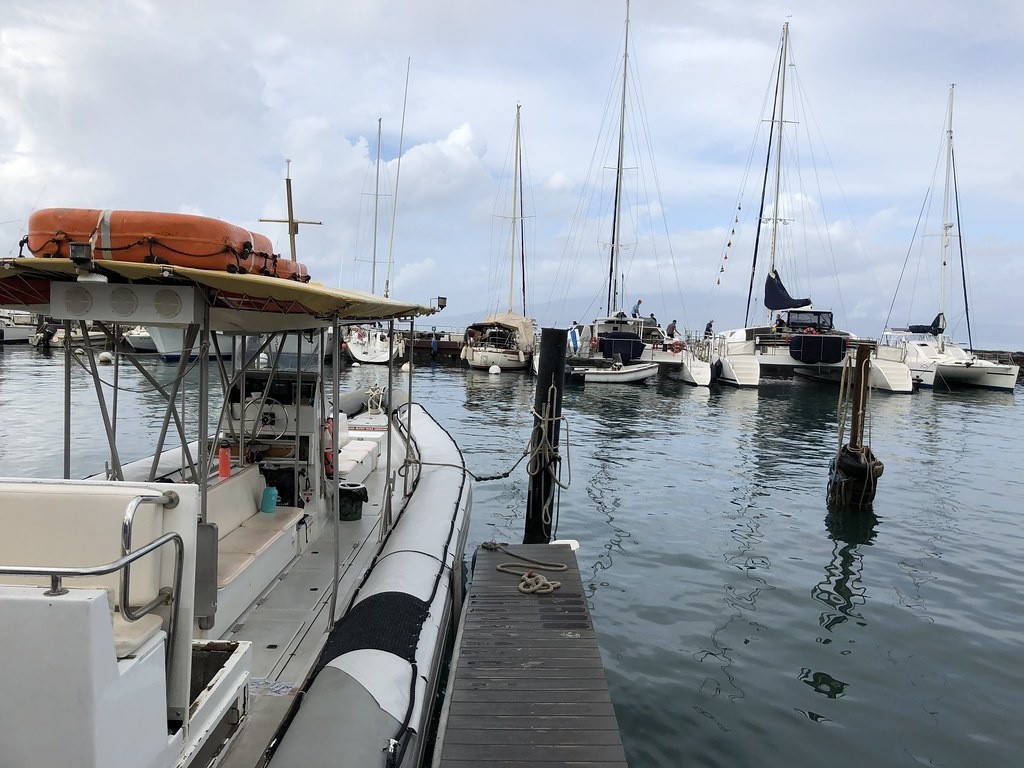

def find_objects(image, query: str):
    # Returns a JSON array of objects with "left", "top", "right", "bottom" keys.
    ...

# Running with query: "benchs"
[
  {"left": 349, "top": 430, "right": 385, "bottom": 458},
  {"left": 206, "top": 463, "right": 304, "bottom": 639},
  {"left": 331, "top": 413, "right": 379, "bottom": 487},
  {"left": 0, "top": 479, "right": 169, "bottom": 768}
]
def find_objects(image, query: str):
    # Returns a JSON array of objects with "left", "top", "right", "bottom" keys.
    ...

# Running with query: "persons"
[
  {"left": 650, "top": 313, "right": 657, "bottom": 325},
  {"left": 667, "top": 320, "right": 681, "bottom": 338},
  {"left": 772, "top": 314, "right": 787, "bottom": 336},
  {"left": 631, "top": 300, "right": 642, "bottom": 332},
  {"left": 704, "top": 320, "right": 714, "bottom": 339}
]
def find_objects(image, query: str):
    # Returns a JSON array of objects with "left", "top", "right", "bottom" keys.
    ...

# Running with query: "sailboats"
[
  {"left": 876, "top": 83, "right": 1020, "bottom": 393},
  {"left": 460, "top": 105, "right": 534, "bottom": 370},
  {"left": 571, "top": 0, "right": 684, "bottom": 368},
  {"left": 704, "top": 21, "right": 856, "bottom": 369},
  {"left": 342, "top": 118, "right": 406, "bottom": 364}
]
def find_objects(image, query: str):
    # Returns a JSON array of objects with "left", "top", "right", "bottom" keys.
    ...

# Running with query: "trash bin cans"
[{"left": 334, "top": 483, "right": 367, "bottom": 520}]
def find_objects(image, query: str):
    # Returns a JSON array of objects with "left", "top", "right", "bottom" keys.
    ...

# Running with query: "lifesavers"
[
  {"left": 276, "top": 256, "right": 308, "bottom": 282},
  {"left": 325, "top": 419, "right": 339, "bottom": 476},
  {"left": 804, "top": 328, "right": 816, "bottom": 335},
  {"left": 30, "top": 209, "right": 276, "bottom": 276},
  {"left": 671, "top": 341, "right": 684, "bottom": 354},
  {"left": 590, "top": 337, "right": 598, "bottom": 348},
  {"left": 845, "top": 338, "right": 850, "bottom": 345}
]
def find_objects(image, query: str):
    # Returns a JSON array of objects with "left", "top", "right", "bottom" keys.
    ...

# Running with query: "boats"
[
  {"left": 146, "top": 326, "right": 233, "bottom": 362},
  {"left": 121, "top": 326, "right": 156, "bottom": 353},
  {"left": 28, "top": 322, "right": 109, "bottom": 348},
  {"left": 0, "top": 316, "right": 38, "bottom": 341},
  {"left": 1, "top": 180, "right": 473, "bottom": 768}
]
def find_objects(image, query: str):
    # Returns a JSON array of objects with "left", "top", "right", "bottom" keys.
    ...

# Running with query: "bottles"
[
  {"left": 219, "top": 440, "right": 231, "bottom": 476},
  {"left": 261, "top": 483, "right": 278, "bottom": 513},
  {"left": 324, "top": 426, "right": 332, "bottom": 452}
]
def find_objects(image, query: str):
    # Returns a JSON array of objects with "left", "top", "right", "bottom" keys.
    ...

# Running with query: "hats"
[
  {"left": 638, "top": 300, "right": 642, "bottom": 303},
  {"left": 776, "top": 314, "right": 780, "bottom": 318},
  {"left": 650, "top": 313, "right": 654, "bottom": 316},
  {"left": 673, "top": 320, "right": 677, "bottom": 322},
  {"left": 710, "top": 320, "right": 714, "bottom": 323}
]
